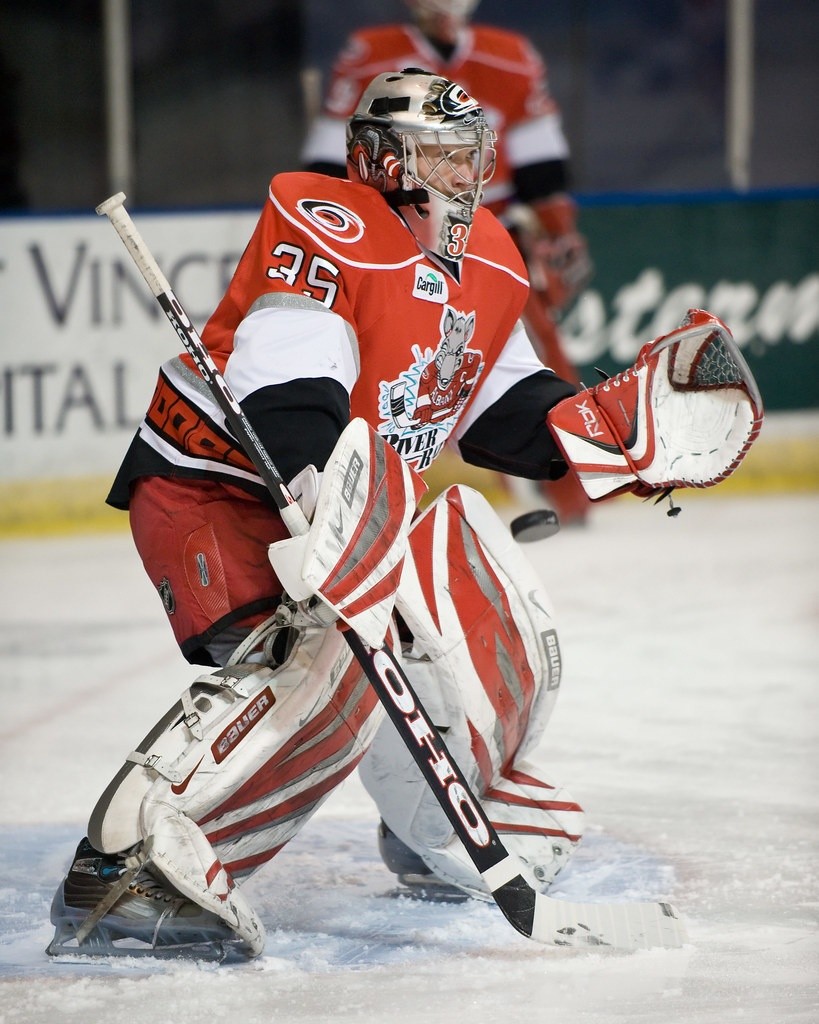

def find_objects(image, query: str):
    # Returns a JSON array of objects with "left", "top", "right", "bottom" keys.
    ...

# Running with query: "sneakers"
[
  {"left": 375, "top": 826, "right": 467, "bottom": 905},
  {"left": 46, "top": 836, "right": 251, "bottom": 968}
]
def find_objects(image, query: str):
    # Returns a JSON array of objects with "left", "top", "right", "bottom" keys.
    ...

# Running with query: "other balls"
[{"left": 510, "top": 509, "right": 561, "bottom": 544}]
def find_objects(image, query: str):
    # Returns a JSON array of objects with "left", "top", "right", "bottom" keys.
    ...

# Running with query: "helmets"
[{"left": 346, "top": 68, "right": 498, "bottom": 262}]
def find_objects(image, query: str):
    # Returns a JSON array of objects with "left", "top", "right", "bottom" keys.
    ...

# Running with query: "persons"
[
  {"left": 300, "top": 0, "right": 598, "bottom": 525},
  {"left": 44, "top": 65, "right": 766, "bottom": 968}
]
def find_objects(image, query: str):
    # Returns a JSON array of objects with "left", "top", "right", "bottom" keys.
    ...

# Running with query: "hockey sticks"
[{"left": 96, "top": 191, "right": 688, "bottom": 952}]
